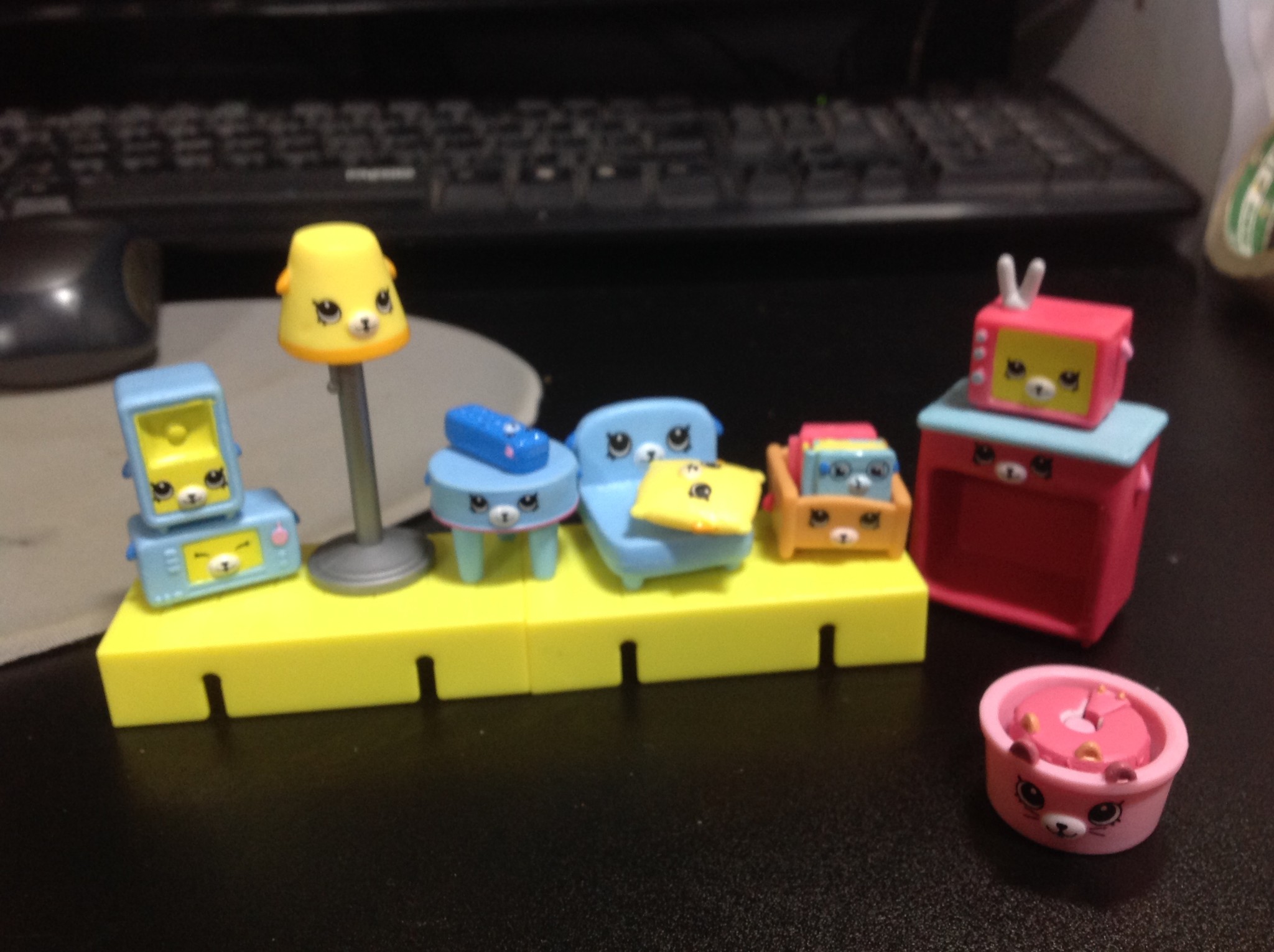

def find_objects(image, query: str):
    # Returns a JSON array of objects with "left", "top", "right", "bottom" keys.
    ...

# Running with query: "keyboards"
[{"left": 3, "top": 70, "right": 1215, "bottom": 233}]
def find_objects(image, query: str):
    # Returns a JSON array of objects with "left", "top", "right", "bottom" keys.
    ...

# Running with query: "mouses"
[{"left": 0, "top": 216, "right": 167, "bottom": 388}]
